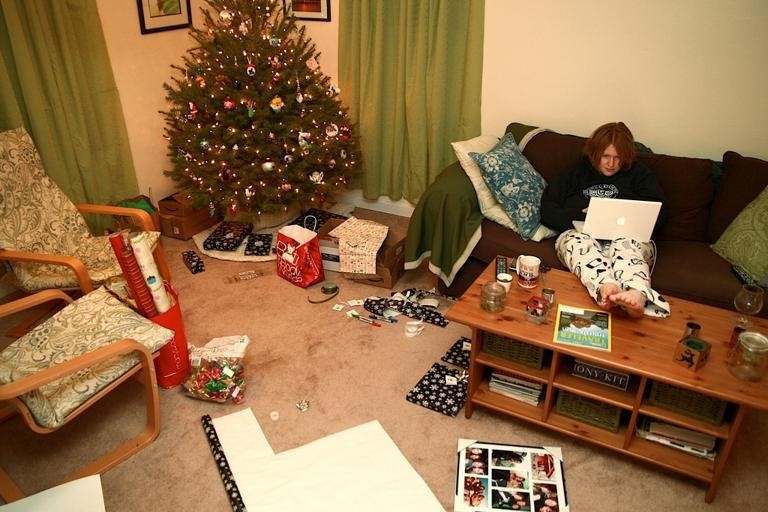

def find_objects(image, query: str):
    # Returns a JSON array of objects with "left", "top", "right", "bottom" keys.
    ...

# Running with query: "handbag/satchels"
[
  {"left": 276, "top": 215, "right": 326, "bottom": 289},
  {"left": 124, "top": 279, "right": 193, "bottom": 390},
  {"left": 110, "top": 187, "right": 163, "bottom": 238}
]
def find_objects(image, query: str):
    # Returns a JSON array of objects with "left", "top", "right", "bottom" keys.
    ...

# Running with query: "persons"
[
  {"left": 541, "top": 121, "right": 674, "bottom": 322},
  {"left": 459, "top": 437, "right": 561, "bottom": 512}
]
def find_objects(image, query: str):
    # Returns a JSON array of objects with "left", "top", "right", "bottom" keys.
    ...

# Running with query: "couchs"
[{"left": 403, "top": 122, "right": 766, "bottom": 318}]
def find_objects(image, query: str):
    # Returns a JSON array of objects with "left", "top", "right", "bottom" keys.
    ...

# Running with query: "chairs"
[
  {"left": 1, "top": 123, "right": 169, "bottom": 296},
  {"left": 1, "top": 282, "right": 176, "bottom": 505}
]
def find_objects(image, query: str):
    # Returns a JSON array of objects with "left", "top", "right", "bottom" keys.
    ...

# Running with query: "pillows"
[
  {"left": 710, "top": 184, "right": 768, "bottom": 294},
  {"left": 466, "top": 131, "right": 551, "bottom": 242}
]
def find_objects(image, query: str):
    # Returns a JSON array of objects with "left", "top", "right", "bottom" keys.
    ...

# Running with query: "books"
[
  {"left": 550, "top": 299, "right": 613, "bottom": 353},
  {"left": 484, "top": 367, "right": 546, "bottom": 406},
  {"left": 636, "top": 415, "right": 719, "bottom": 464}
]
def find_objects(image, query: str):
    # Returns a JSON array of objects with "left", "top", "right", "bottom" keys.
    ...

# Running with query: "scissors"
[{"left": 539, "top": 266, "right": 551, "bottom": 283}]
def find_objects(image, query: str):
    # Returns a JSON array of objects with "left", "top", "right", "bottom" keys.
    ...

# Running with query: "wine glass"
[{"left": 730, "top": 285, "right": 764, "bottom": 328}]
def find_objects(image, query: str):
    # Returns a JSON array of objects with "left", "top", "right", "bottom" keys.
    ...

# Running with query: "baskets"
[
  {"left": 481, "top": 329, "right": 545, "bottom": 371},
  {"left": 554, "top": 387, "right": 622, "bottom": 434},
  {"left": 646, "top": 380, "right": 730, "bottom": 427}
]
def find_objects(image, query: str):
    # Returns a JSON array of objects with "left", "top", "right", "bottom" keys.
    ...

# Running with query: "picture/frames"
[
  {"left": 283, "top": 0, "right": 332, "bottom": 23},
  {"left": 134, "top": 0, "right": 194, "bottom": 35}
]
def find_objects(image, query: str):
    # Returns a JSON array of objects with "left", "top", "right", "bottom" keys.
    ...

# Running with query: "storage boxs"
[
  {"left": 337, "top": 207, "right": 416, "bottom": 292},
  {"left": 158, "top": 211, "right": 219, "bottom": 242},
  {"left": 316, "top": 216, "right": 352, "bottom": 274},
  {"left": 157, "top": 187, "right": 208, "bottom": 215}
]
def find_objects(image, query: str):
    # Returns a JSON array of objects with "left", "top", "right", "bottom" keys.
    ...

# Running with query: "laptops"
[{"left": 571, "top": 196, "right": 663, "bottom": 240}]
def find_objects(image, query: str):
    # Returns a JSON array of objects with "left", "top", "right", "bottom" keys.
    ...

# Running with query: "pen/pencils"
[
  {"left": 369, "top": 315, "right": 398, "bottom": 323},
  {"left": 352, "top": 315, "right": 382, "bottom": 327}
]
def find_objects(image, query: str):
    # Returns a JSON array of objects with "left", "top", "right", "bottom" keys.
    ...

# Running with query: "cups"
[
  {"left": 682, "top": 322, "right": 702, "bottom": 338},
  {"left": 526, "top": 296, "right": 550, "bottom": 325},
  {"left": 515, "top": 255, "right": 541, "bottom": 289},
  {"left": 496, "top": 273, "right": 513, "bottom": 294},
  {"left": 480, "top": 283, "right": 506, "bottom": 313},
  {"left": 541, "top": 288, "right": 555, "bottom": 309}
]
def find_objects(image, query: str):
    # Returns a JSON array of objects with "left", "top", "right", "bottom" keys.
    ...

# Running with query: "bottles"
[
  {"left": 495, "top": 256, "right": 507, "bottom": 278},
  {"left": 726, "top": 330, "right": 768, "bottom": 381}
]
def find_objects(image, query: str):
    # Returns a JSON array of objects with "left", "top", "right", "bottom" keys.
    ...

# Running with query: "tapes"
[{"left": 404, "top": 326, "right": 417, "bottom": 338}]
